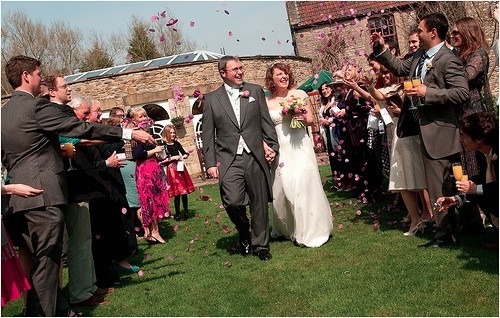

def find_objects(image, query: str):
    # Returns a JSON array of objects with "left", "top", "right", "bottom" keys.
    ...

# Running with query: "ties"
[
  {"left": 412, "top": 55, "right": 429, "bottom": 121},
  {"left": 484, "top": 152, "right": 499, "bottom": 231}
]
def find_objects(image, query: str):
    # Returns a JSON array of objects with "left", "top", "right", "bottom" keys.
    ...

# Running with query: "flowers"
[
  {"left": 279, "top": 95, "right": 306, "bottom": 128},
  {"left": 240, "top": 89, "right": 250, "bottom": 98}
]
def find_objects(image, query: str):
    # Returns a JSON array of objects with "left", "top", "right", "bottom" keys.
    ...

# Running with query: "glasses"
[
  {"left": 114, "top": 114, "right": 125, "bottom": 118},
  {"left": 380, "top": 70, "right": 391, "bottom": 75},
  {"left": 450, "top": 30, "right": 459, "bottom": 36},
  {"left": 48, "top": 84, "right": 68, "bottom": 90}
]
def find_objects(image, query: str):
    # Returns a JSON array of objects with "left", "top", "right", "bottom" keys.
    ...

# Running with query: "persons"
[
  {"left": 444, "top": 33, "right": 459, "bottom": 53},
  {"left": 155, "top": 125, "right": 196, "bottom": 221},
  {"left": 439, "top": 17, "right": 496, "bottom": 233},
  {"left": 204, "top": 56, "right": 280, "bottom": 261},
  {"left": 0, "top": 55, "right": 172, "bottom": 317},
  {"left": 434, "top": 111, "right": 498, "bottom": 254},
  {"left": 316, "top": 37, "right": 437, "bottom": 239},
  {"left": 407, "top": 29, "right": 422, "bottom": 52},
  {"left": 262, "top": 61, "right": 335, "bottom": 248},
  {"left": 369, "top": 13, "right": 481, "bottom": 249},
  {"left": 492, "top": 4, "right": 499, "bottom": 70}
]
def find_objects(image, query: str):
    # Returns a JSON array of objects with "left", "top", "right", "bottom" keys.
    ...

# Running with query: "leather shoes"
[
  {"left": 417, "top": 218, "right": 484, "bottom": 248},
  {"left": 72, "top": 287, "right": 115, "bottom": 307},
  {"left": 239, "top": 237, "right": 273, "bottom": 261},
  {"left": 481, "top": 235, "right": 498, "bottom": 250}
]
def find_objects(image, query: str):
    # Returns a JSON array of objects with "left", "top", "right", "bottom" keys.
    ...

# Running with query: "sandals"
[{"left": 155, "top": 209, "right": 188, "bottom": 228}]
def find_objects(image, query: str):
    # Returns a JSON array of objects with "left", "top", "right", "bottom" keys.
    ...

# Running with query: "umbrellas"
[{"left": 297, "top": 65, "right": 339, "bottom": 95}]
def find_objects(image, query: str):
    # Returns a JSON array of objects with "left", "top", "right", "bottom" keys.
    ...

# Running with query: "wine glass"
[
  {"left": 460, "top": 171, "right": 470, "bottom": 202},
  {"left": 412, "top": 77, "right": 424, "bottom": 106},
  {"left": 404, "top": 79, "right": 418, "bottom": 110},
  {"left": 64, "top": 142, "right": 77, "bottom": 171},
  {"left": 453, "top": 162, "right": 464, "bottom": 196}
]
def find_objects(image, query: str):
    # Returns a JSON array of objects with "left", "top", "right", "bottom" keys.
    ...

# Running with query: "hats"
[{"left": 326, "top": 70, "right": 349, "bottom": 85}]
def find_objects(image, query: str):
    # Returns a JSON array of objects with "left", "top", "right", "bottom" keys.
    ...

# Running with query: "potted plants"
[{"left": 171, "top": 116, "right": 185, "bottom": 129}]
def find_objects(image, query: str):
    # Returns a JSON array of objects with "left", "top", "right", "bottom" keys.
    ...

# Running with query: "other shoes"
[
  {"left": 330, "top": 173, "right": 400, "bottom": 211},
  {"left": 62, "top": 309, "right": 85, "bottom": 317}
]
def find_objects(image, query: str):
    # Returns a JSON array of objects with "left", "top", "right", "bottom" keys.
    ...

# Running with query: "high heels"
[
  {"left": 401, "top": 208, "right": 436, "bottom": 236},
  {"left": 144, "top": 233, "right": 167, "bottom": 245},
  {"left": 113, "top": 260, "right": 140, "bottom": 274}
]
